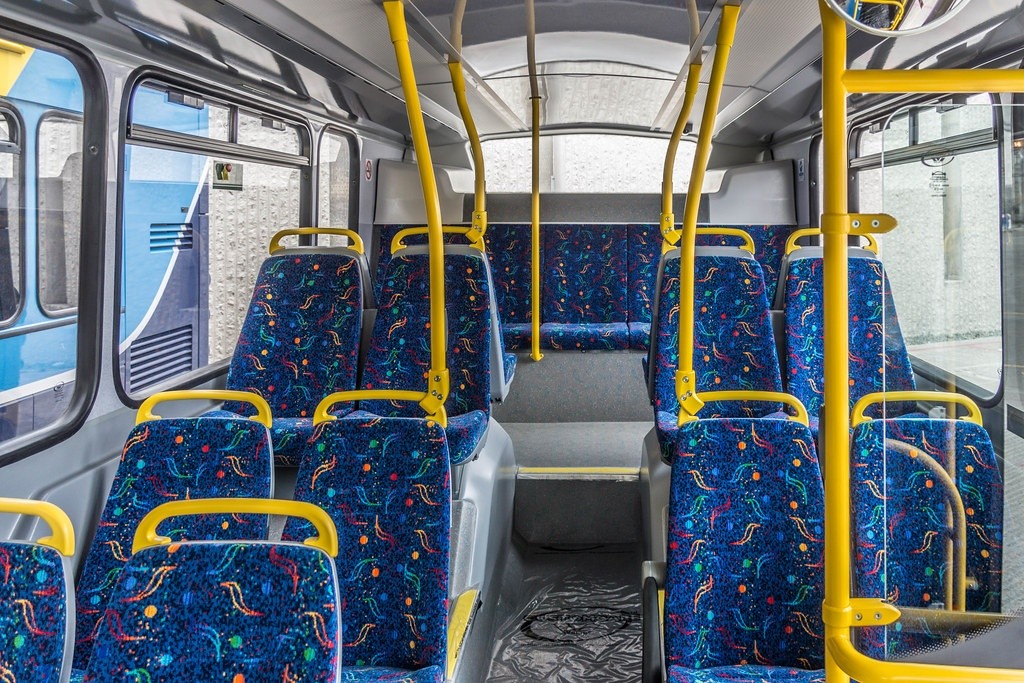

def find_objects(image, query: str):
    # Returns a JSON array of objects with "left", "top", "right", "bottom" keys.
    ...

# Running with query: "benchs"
[{"left": 1, "top": 221, "right": 1024, "bottom": 683}]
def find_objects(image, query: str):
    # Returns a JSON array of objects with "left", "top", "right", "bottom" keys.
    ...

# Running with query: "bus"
[{"left": 1, "top": 39, "right": 211, "bottom": 441}]
